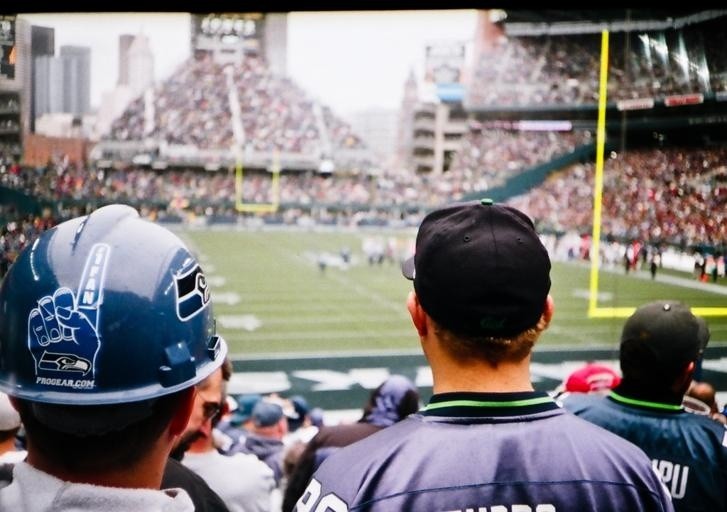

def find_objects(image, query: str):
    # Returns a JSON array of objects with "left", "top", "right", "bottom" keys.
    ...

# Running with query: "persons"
[
  {"left": 0, "top": 204, "right": 227, "bottom": 511},
  {"left": 160, "top": 357, "right": 232, "bottom": 512},
  {"left": 280, "top": 375, "right": 420, "bottom": 511},
  {"left": 292, "top": 198, "right": 673, "bottom": 512},
  {"left": 181, "top": 380, "right": 278, "bottom": 511},
  {"left": 0, "top": 365, "right": 726, "bottom": 468},
  {"left": 0, "top": 16, "right": 726, "bottom": 281},
  {"left": 559, "top": 300, "right": 726, "bottom": 511}
]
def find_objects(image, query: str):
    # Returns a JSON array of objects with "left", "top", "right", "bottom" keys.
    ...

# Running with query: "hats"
[
  {"left": 620, "top": 301, "right": 708, "bottom": 361},
  {"left": 252, "top": 402, "right": 282, "bottom": 427},
  {"left": 403, "top": 198, "right": 551, "bottom": 336}
]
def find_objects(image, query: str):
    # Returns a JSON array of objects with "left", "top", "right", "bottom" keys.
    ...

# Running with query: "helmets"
[{"left": 0, "top": 203, "right": 227, "bottom": 406}]
